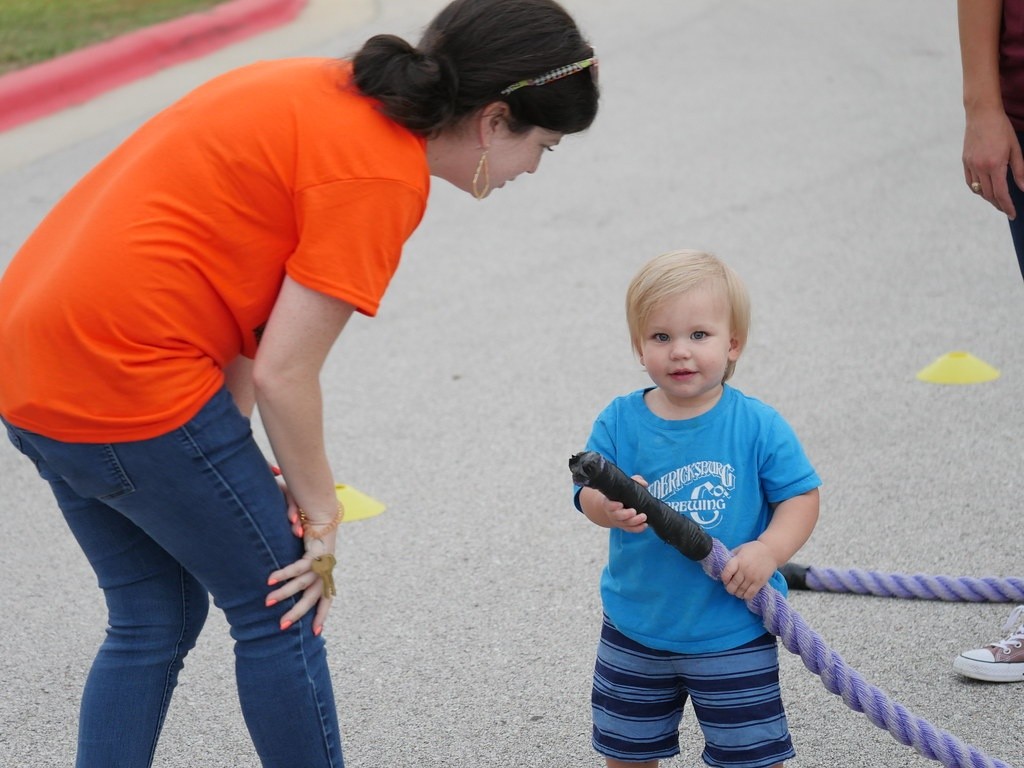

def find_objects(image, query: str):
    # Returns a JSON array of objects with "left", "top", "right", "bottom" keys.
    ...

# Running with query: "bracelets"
[{"left": 299, "top": 500, "right": 343, "bottom": 537}]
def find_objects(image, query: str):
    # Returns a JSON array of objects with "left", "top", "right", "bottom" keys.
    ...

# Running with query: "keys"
[{"left": 312, "top": 553, "right": 336, "bottom": 597}]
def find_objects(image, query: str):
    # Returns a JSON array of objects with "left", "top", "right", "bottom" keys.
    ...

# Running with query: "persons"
[
  {"left": 0, "top": 1, "right": 599, "bottom": 768},
  {"left": 954, "top": 0, "right": 1023, "bottom": 684},
  {"left": 575, "top": 251, "right": 823, "bottom": 768}
]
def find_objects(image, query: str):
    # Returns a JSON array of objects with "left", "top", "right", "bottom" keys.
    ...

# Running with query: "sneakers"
[{"left": 953, "top": 605, "right": 1024, "bottom": 682}]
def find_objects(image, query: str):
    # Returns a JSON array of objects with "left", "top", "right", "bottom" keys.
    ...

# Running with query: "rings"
[{"left": 972, "top": 182, "right": 981, "bottom": 192}]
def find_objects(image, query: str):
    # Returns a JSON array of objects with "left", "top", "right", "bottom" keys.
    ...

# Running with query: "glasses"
[{"left": 501, "top": 46, "right": 601, "bottom": 94}]
